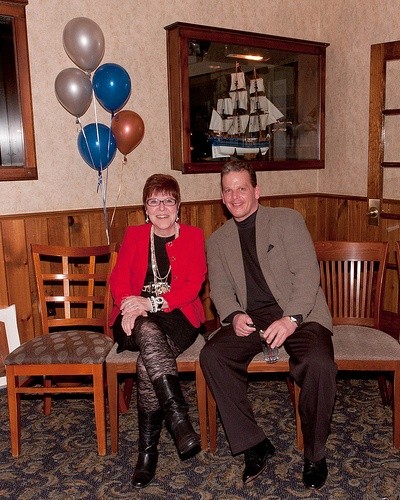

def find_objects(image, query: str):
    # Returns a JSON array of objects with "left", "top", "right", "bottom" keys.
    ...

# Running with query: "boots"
[
  {"left": 131, "top": 407, "right": 163, "bottom": 488},
  {"left": 152, "top": 374, "right": 202, "bottom": 461}
]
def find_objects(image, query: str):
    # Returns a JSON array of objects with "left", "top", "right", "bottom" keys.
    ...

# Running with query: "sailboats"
[{"left": 206, "top": 58, "right": 292, "bottom": 161}]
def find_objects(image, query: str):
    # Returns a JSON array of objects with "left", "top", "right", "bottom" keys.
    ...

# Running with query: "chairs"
[{"left": 5, "top": 242, "right": 400, "bottom": 457}]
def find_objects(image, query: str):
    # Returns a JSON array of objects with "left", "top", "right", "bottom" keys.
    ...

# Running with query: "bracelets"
[{"left": 147, "top": 296, "right": 157, "bottom": 313}]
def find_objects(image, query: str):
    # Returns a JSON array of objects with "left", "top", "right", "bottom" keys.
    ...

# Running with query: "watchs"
[
  {"left": 288, "top": 315, "right": 302, "bottom": 327},
  {"left": 155, "top": 297, "right": 163, "bottom": 311}
]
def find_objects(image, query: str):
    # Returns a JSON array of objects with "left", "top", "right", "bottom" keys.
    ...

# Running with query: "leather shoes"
[
  {"left": 303, "top": 456, "right": 328, "bottom": 490},
  {"left": 243, "top": 438, "right": 276, "bottom": 484}
]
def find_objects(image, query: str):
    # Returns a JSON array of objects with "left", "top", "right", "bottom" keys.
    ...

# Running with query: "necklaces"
[{"left": 150, "top": 222, "right": 179, "bottom": 296}]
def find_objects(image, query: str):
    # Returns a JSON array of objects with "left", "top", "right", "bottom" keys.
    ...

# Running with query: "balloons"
[
  {"left": 111, "top": 110, "right": 145, "bottom": 156},
  {"left": 63, "top": 17, "right": 105, "bottom": 72},
  {"left": 55, "top": 67, "right": 94, "bottom": 118},
  {"left": 92, "top": 63, "right": 131, "bottom": 114},
  {"left": 77, "top": 123, "right": 116, "bottom": 171}
]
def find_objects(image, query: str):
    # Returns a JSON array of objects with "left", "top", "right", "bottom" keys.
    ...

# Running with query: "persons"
[
  {"left": 199, "top": 158, "right": 336, "bottom": 490},
  {"left": 109, "top": 173, "right": 209, "bottom": 489}
]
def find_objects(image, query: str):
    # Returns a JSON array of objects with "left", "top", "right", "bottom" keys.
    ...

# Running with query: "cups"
[{"left": 259, "top": 330, "right": 278, "bottom": 362}]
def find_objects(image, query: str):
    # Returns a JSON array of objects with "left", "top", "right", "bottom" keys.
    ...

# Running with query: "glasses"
[{"left": 147, "top": 197, "right": 176, "bottom": 205}]
